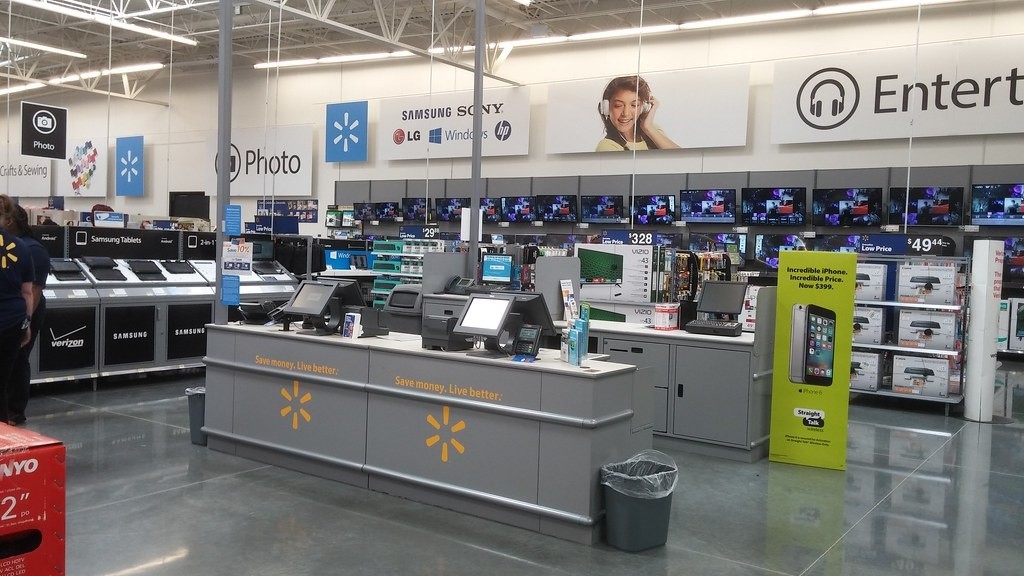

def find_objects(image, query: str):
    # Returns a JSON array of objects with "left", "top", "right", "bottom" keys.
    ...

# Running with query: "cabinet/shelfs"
[
  {"left": 603, "top": 337, "right": 752, "bottom": 453},
  {"left": 851, "top": 253, "right": 973, "bottom": 417}
]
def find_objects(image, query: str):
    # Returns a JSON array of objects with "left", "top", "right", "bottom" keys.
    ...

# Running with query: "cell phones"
[{"left": 789, "top": 304, "right": 836, "bottom": 386}]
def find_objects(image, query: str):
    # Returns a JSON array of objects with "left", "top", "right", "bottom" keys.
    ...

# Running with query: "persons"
[
  {"left": 0, "top": 195, "right": 51, "bottom": 424},
  {"left": 594, "top": 76, "right": 681, "bottom": 152}
]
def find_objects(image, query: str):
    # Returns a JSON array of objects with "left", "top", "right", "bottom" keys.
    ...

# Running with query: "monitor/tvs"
[
  {"left": 283, "top": 279, "right": 340, "bottom": 317},
  {"left": 354, "top": 202, "right": 399, "bottom": 222},
  {"left": 436, "top": 183, "right": 1024, "bottom": 339},
  {"left": 402, "top": 198, "right": 431, "bottom": 221},
  {"left": 317, "top": 277, "right": 367, "bottom": 307},
  {"left": 578, "top": 248, "right": 623, "bottom": 289}
]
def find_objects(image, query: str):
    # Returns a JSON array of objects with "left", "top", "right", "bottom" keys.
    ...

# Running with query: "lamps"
[
  {"left": 10, "top": 0, "right": 198, "bottom": 48},
  {"left": 251, "top": 0, "right": 959, "bottom": 73},
  {"left": 0, "top": 36, "right": 87, "bottom": 59},
  {"left": 0, "top": 63, "right": 165, "bottom": 96}
]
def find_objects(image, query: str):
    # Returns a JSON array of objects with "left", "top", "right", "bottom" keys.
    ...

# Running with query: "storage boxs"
[
  {"left": 991, "top": 297, "right": 1024, "bottom": 351},
  {"left": 851, "top": 307, "right": 886, "bottom": 344},
  {"left": 892, "top": 353, "right": 951, "bottom": 397},
  {"left": 855, "top": 263, "right": 888, "bottom": 300},
  {"left": 850, "top": 350, "right": 883, "bottom": 391},
  {"left": 897, "top": 264, "right": 958, "bottom": 305},
  {"left": 0, "top": 422, "right": 68, "bottom": 576},
  {"left": 897, "top": 310, "right": 957, "bottom": 353}
]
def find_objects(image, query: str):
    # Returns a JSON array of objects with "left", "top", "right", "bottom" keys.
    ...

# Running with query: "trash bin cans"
[
  {"left": 602, "top": 459, "right": 678, "bottom": 552},
  {"left": 186, "top": 387, "right": 207, "bottom": 446}
]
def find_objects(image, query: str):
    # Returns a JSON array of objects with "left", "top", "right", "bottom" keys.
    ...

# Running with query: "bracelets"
[{"left": 26, "top": 314, "right": 33, "bottom": 321}]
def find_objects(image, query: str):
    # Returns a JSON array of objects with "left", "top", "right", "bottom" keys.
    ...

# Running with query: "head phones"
[{"left": 600, "top": 77, "right": 651, "bottom": 115}]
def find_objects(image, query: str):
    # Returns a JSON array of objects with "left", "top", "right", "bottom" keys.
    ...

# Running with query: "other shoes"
[{"left": 8, "top": 410, "right": 26, "bottom": 420}]
